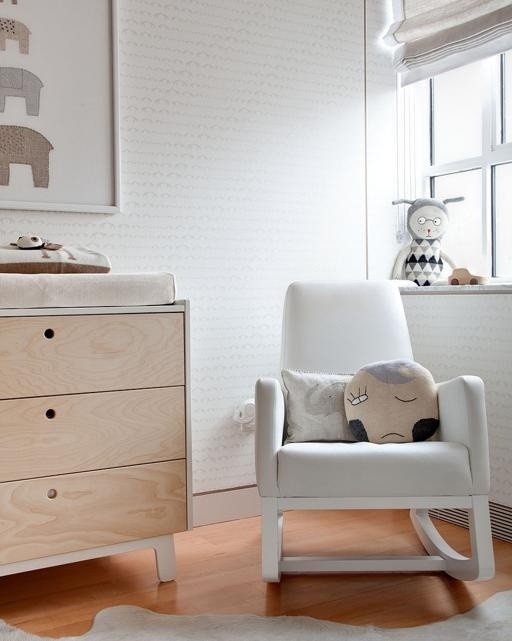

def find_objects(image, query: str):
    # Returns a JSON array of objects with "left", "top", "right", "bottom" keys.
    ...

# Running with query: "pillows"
[{"left": 281, "top": 359, "right": 438, "bottom": 442}]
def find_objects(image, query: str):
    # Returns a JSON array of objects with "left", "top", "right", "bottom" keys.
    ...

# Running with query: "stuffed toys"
[
  {"left": 10, "top": 235, "right": 47, "bottom": 249},
  {"left": 390, "top": 194, "right": 463, "bottom": 287}
]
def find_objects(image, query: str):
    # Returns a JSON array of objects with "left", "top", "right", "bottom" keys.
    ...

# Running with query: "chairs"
[{"left": 255, "top": 281, "right": 498, "bottom": 582}]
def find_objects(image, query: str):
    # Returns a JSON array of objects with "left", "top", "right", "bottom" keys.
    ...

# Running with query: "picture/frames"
[{"left": 1, "top": 0, "right": 122, "bottom": 215}]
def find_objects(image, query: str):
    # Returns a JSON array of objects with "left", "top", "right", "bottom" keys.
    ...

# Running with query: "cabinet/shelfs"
[{"left": 2, "top": 300, "right": 194, "bottom": 582}]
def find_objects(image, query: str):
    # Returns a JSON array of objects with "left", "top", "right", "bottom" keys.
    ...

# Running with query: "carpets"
[{"left": 1, "top": 587, "right": 512, "bottom": 641}]
